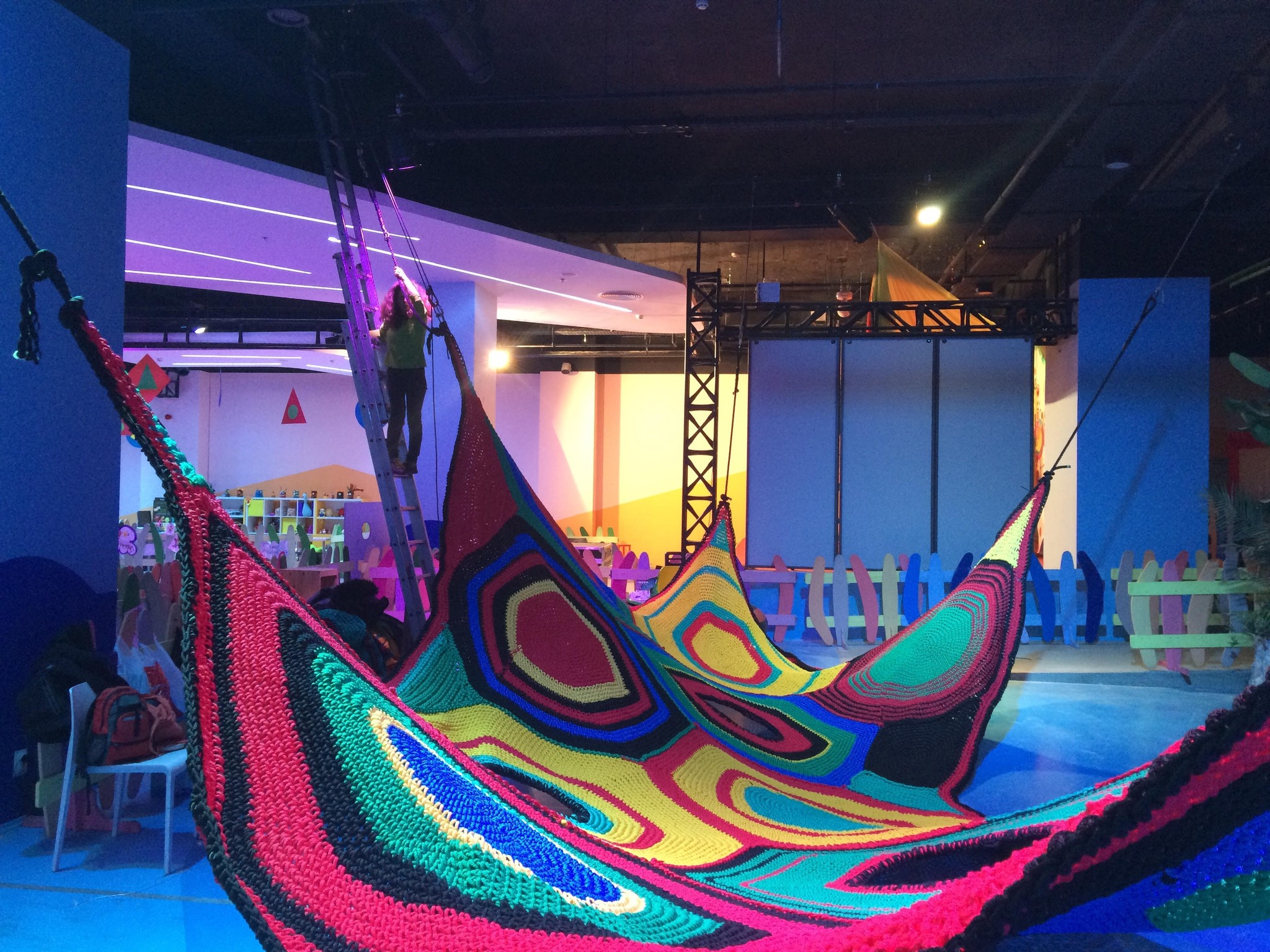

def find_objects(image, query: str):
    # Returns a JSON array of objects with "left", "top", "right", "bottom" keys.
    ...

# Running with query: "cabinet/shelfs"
[{"left": 215, "top": 497, "right": 364, "bottom": 556}]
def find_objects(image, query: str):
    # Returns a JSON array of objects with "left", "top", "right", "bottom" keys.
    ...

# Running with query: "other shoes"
[
  {"left": 403, "top": 460, "right": 418, "bottom": 475},
  {"left": 390, "top": 458, "right": 409, "bottom": 477}
]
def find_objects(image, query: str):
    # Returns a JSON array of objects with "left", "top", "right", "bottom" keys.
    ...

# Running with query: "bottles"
[{"left": 295, "top": 548, "right": 302, "bottom": 561}]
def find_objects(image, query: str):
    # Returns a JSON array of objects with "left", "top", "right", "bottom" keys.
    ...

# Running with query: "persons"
[{"left": 367, "top": 266, "right": 434, "bottom": 475}]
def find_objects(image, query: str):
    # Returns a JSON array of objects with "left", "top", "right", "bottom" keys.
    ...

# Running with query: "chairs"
[{"left": 51, "top": 681, "right": 187, "bottom": 877}]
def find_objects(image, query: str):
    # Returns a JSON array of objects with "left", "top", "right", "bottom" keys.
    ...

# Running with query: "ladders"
[{"left": 297, "top": 19, "right": 439, "bottom": 646}]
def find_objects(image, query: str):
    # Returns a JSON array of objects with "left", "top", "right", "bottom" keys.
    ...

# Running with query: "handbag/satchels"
[{"left": 141, "top": 695, "right": 183, "bottom": 755}]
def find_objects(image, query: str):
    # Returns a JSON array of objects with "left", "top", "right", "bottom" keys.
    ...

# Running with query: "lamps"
[
  {"left": 974, "top": 280, "right": 994, "bottom": 296},
  {"left": 560, "top": 361, "right": 572, "bottom": 375}
]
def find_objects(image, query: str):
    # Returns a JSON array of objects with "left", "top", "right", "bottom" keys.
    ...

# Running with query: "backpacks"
[{"left": 80, "top": 674, "right": 155, "bottom": 766}]
